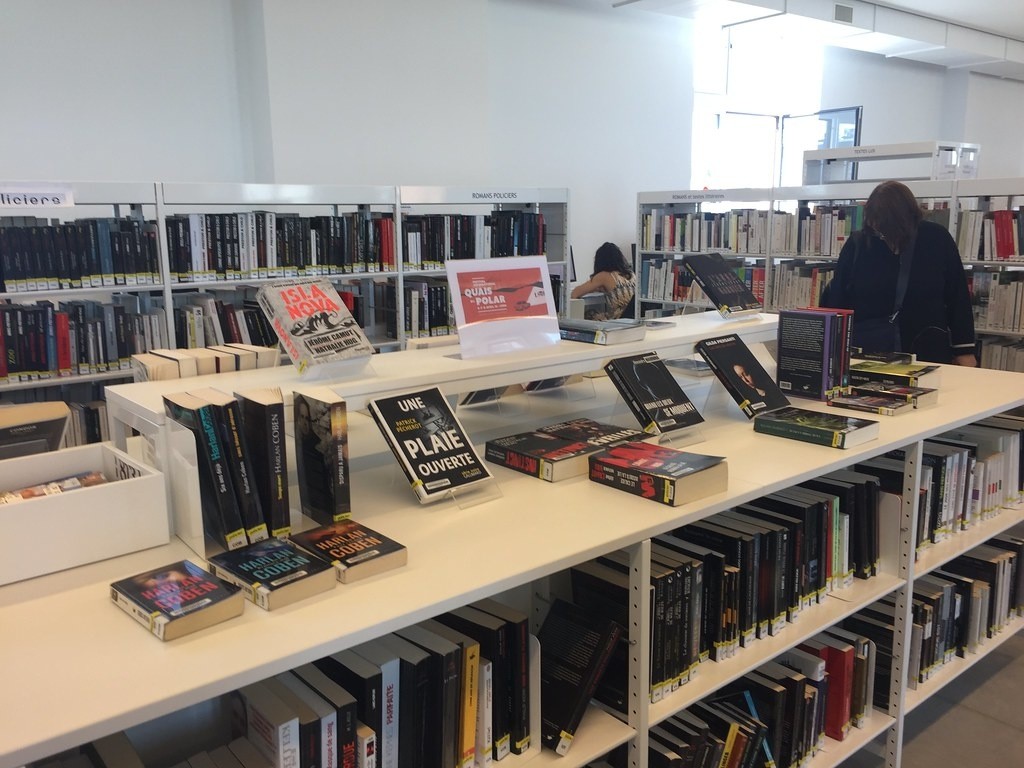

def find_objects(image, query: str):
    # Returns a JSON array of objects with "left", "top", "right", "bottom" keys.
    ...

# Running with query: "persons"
[
  {"left": 295, "top": 400, "right": 311, "bottom": 436},
  {"left": 733, "top": 362, "right": 766, "bottom": 398},
  {"left": 571, "top": 242, "right": 636, "bottom": 320},
  {"left": 820, "top": 181, "right": 977, "bottom": 368}
]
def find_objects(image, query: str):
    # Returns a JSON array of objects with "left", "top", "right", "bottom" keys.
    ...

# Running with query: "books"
[
  {"left": 641, "top": 204, "right": 1024, "bottom": 373},
  {"left": 695, "top": 333, "right": 791, "bottom": 421},
  {"left": 110, "top": 386, "right": 408, "bottom": 643},
  {"left": 178, "top": 414, "right": 1024, "bottom": 768},
  {"left": 558, "top": 319, "right": 645, "bottom": 345},
  {"left": 458, "top": 386, "right": 508, "bottom": 406},
  {"left": 603, "top": 351, "right": 705, "bottom": 441},
  {"left": 521, "top": 375, "right": 570, "bottom": 392},
  {"left": 753, "top": 406, "right": 880, "bottom": 449},
  {"left": 681, "top": 253, "right": 763, "bottom": 317},
  {"left": 367, "top": 386, "right": 495, "bottom": 505},
  {"left": 776, "top": 307, "right": 939, "bottom": 416},
  {"left": 0, "top": 401, "right": 71, "bottom": 451},
  {"left": 0, "top": 209, "right": 547, "bottom": 450},
  {"left": 639, "top": 321, "right": 676, "bottom": 330}
]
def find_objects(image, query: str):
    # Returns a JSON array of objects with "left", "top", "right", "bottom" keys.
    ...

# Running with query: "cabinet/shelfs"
[
  {"left": 633, "top": 177, "right": 1023, "bottom": 336},
  {"left": 1, "top": 183, "right": 573, "bottom": 393},
  {"left": 0, "top": 310, "right": 1024, "bottom": 768}
]
